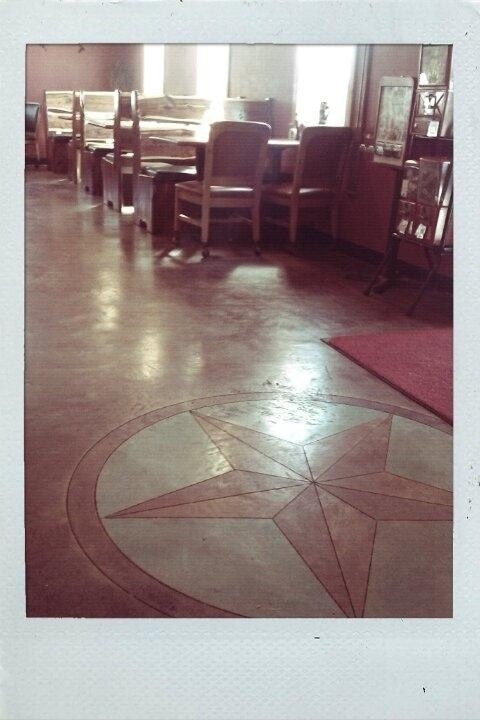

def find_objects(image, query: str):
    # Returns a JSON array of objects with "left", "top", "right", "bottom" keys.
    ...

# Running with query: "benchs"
[
  {"left": 102, "top": 89, "right": 116, "bottom": 214},
  {"left": 45, "top": 91, "right": 75, "bottom": 173},
  {"left": 116, "top": 90, "right": 131, "bottom": 213},
  {"left": 65, "top": 92, "right": 80, "bottom": 187},
  {"left": 80, "top": 89, "right": 116, "bottom": 195},
  {"left": 131, "top": 91, "right": 272, "bottom": 235}
]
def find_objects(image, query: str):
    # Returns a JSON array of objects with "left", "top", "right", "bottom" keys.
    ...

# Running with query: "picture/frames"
[{"left": 375, "top": 74, "right": 415, "bottom": 148}]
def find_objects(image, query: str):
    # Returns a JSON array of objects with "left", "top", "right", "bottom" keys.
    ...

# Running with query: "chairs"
[
  {"left": 26, "top": 103, "right": 39, "bottom": 167},
  {"left": 171, "top": 120, "right": 272, "bottom": 257},
  {"left": 260, "top": 127, "right": 357, "bottom": 242}
]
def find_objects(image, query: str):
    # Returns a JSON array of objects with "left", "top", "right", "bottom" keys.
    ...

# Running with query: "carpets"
[{"left": 321, "top": 328, "right": 453, "bottom": 425}]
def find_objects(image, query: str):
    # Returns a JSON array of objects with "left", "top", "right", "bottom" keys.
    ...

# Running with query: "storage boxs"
[{"left": 392, "top": 157, "right": 453, "bottom": 246}]
